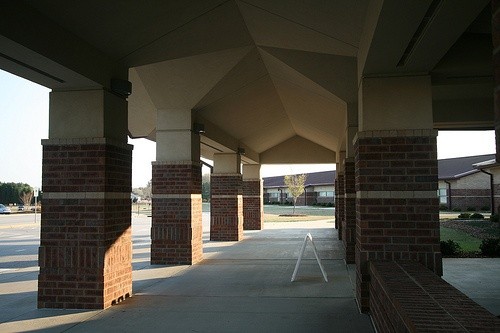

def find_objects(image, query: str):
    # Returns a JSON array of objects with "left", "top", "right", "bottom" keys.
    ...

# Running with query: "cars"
[
  {"left": 0, "top": 204, "right": 11, "bottom": 214},
  {"left": 18, "top": 206, "right": 24, "bottom": 211}
]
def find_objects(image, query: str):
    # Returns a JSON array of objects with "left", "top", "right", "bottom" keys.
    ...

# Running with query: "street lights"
[{"left": 471, "top": 164, "right": 494, "bottom": 219}]
